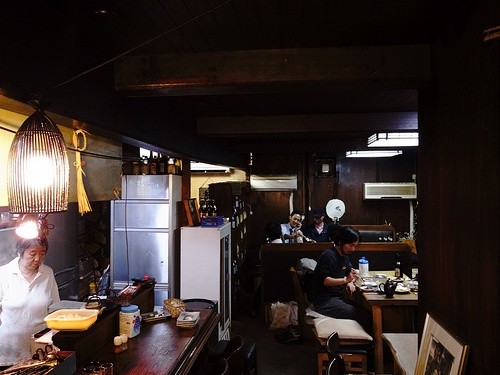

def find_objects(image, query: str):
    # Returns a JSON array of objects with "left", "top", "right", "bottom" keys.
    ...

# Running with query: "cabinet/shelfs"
[{"left": 207, "top": 182, "right": 257, "bottom": 319}]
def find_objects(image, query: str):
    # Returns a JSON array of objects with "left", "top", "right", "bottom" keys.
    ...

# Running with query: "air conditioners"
[
  {"left": 190, "top": 162, "right": 234, "bottom": 174},
  {"left": 249, "top": 174, "right": 298, "bottom": 192},
  {"left": 361, "top": 182, "right": 417, "bottom": 199}
]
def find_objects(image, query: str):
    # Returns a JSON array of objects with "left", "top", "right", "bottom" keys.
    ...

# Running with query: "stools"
[{"left": 218, "top": 340, "right": 257, "bottom": 375}]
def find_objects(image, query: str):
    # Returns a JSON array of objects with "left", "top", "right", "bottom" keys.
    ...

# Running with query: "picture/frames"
[{"left": 414, "top": 311, "right": 472, "bottom": 375}]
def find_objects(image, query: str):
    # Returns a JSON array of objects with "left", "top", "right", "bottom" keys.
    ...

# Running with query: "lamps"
[
  {"left": 6, "top": 101, "right": 73, "bottom": 240},
  {"left": 366, "top": 130, "right": 420, "bottom": 148},
  {"left": 343, "top": 149, "right": 402, "bottom": 160}
]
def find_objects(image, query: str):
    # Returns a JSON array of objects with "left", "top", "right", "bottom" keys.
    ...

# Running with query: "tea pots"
[{"left": 379, "top": 278, "right": 399, "bottom": 298}]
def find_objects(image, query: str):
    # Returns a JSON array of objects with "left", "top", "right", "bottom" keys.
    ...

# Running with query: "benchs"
[
  {"left": 258, "top": 241, "right": 416, "bottom": 320},
  {"left": 343, "top": 222, "right": 397, "bottom": 241}
]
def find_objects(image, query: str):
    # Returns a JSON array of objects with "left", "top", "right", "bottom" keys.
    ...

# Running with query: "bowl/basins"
[{"left": 44, "top": 309, "right": 99, "bottom": 332}]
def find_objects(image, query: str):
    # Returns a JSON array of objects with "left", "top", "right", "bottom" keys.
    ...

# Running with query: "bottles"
[
  {"left": 119, "top": 305, "right": 142, "bottom": 338},
  {"left": 199, "top": 196, "right": 247, "bottom": 294},
  {"left": 114, "top": 334, "right": 129, "bottom": 353},
  {"left": 122, "top": 155, "right": 182, "bottom": 175},
  {"left": 359, "top": 256, "right": 369, "bottom": 275},
  {"left": 395, "top": 255, "right": 403, "bottom": 278}
]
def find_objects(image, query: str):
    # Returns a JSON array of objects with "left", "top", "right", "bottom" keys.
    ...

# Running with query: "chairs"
[
  {"left": 215, "top": 335, "right": 247, "bottom": 375},
  {"left": 325, "top": 332, "right": 350, "bottom": 375},
  {"left": 289, "top": 265, "right": 372, "bottom": 375}
]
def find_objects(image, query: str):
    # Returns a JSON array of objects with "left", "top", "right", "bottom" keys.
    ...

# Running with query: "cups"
[{"left": 81, "top": 361, "right": 114, "bottom": 375}]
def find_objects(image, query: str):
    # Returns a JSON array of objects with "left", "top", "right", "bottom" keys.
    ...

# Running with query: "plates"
[
  {"left": 177, "top": 311, "right": 201, "bottom": 328},
  {"left": 359, "top": 274, "right": 418, "bottom": 293}
]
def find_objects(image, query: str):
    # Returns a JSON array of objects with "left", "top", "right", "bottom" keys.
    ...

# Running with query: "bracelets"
[{"left": 344, "top": 277, "right": 346, "bottom": 283}]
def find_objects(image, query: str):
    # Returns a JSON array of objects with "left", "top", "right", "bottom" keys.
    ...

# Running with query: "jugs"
[{"left": 84, "top": 295, "right": 106, "bottom": 315}]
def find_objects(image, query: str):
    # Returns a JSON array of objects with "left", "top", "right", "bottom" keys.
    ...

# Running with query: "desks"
[
  {"left": 80, "top": 308, "right": 221, "bottom": 375},
  {"left": 353, "top": 270, "right": 418, "bottom": 375},
  {"left": 380, "top": 330, "right": 418, "bottom": 375}
]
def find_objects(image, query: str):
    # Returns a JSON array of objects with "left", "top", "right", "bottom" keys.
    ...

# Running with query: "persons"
[
  {"left": 326, "top": 224, "right": 341, "bottom": 241},
  {"left": 304, "top": 208, "right": 328, "bottom": 242},
  {"left": 266, "top": 221, "right": 283, "bottom": 244},
  {"left": 312, "top": 226, "right": 371, "bottom": 329},
  {"left": 0, "top": 236, "right": 59, "bottom": 375},
  {"left": 280, "top": 210, "right": 304, "bottom": 244}
]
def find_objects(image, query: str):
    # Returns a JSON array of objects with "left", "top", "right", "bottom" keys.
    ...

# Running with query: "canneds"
[
  {"left": 131, "top": 161, "right": 176, "bottom": 174},
  {"left": 119, "top": 305, "right": 140, "bottom": 338}
]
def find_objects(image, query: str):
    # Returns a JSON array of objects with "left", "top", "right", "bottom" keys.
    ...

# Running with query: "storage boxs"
[{"left": 44, "top": 313, "right": 101, "bottom": 330}]
